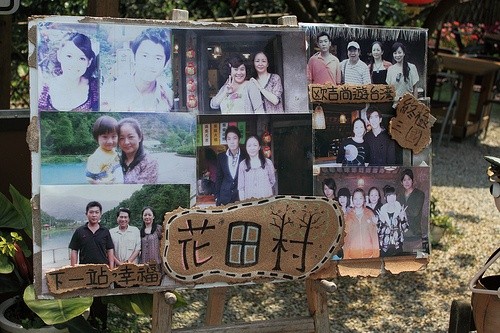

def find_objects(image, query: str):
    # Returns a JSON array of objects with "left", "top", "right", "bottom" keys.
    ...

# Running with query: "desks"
[{"left": 434, "top": 52, "right": 500, "bottom": 142}]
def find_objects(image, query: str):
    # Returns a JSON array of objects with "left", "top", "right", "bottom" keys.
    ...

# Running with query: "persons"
[
  {"left": 385, "top": 41, "right": 420, "bottom": 99},
  {"left": 142, "top": 206, "right": 165, "bottom": 264},
  {"left": 366, "top": 186, "right": 384, "bottom": 213},
  {"left": 342, "top": 40, "right": 372, "bottom": 94},
  {"left": 343, "top": 187, "right": 380, "bottom": 258},
  {"left": 336, "top": 117, "right": 370, "bottom": 167},
  {"left": 116, "top": 118, "right": 160, "bottom": 185},
  {"left": 337, "top": 186, "right": 352, "bottom": 209},
  {"left": 397, "top": 168, "right": 425, "bottom": 251},
  {"left": 209, "top": 51, "right": 266, "bottom": 114},
  {"left": 71, "top": 200, "right": 115, "bottom": 269},
  {"left": 251, "top": 46, "right": 284, "bottom": 114},
  {"left": 83, "top": 115, "right": 124, "bottom": 185},
  {"left": 236, "top": 133, "right": 276, "bottom": 201},
  {"left": 212, "top": 125, "right": 248, "bottom": 208},
  {"left": 100, "top": 27, "right": 173, "bottom": 113},
  {"left": 364, "top": 104, "right": 396, "bottom": 166},
  {"left": 308, "top": 31, "right": 342, "bottom": 96},
  {"left": 321, "top": 176, "right": 338, "bottom": 200},
  {"left": 109, "top": 206, "right": 142, "bottom": 265},
  {"left": 368, "top": 41, "right": 393, "bottom": 84},
  {"left": 378, "top": 184, "right": 408, "bottom": 253},
  {"left": 38, "top": 31, "right": 99, "bottom": 112}
]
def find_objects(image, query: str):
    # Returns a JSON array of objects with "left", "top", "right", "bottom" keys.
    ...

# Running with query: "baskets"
[{"left": 312, "top": 106, "right": 325, "bottom": 129}]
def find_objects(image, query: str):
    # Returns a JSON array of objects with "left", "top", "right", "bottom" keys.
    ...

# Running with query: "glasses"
[
  {"left": 118, "top": 207, "right": 130, "bottom": 213},
  {"left": 396, "top": 73, "right": 401, "bottom": 82}
]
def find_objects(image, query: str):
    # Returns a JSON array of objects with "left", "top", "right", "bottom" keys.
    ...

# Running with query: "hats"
[{"left": 347, "top": 41, "right": 360, "bottom": 51}]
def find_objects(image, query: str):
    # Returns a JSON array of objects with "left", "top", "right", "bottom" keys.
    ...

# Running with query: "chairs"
[{"left": 427, "top": 48, "right": 500, "bottom": 148}]
